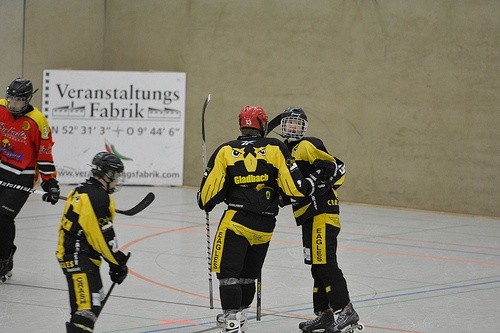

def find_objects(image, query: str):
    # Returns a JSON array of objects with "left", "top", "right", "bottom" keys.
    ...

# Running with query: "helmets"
[
  {"left": 6, "top": 77, "right": 33, "bottom": 98},
  {"left": 282, "top": 107, "right": 309, "bottom": 122},
  {"left": 91, "top": 152, "right": 124, "bottom": 178},
  {"left": 239, "top": 104, "right": 269, "bottom": 130}
]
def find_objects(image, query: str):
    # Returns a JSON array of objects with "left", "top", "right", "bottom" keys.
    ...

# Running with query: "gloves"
[
  {"left": 40, "top": 178, "right": 60, "bottom": 205},
  {"left": 108, "top": 264, "right": 128, "bottom": 285}
]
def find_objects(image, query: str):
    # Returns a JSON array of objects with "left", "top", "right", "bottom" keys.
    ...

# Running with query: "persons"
[
  {"left": 0, "top": 78, "right": 60, "bottom": 276},
  {"left": 278, "top": 107, "right": 364, "bottom": 333},
  {"left": 197, "top": 106, "right": 326, "bottom": 333},
  {"left": 55, "top": 151, "right": 128, "bottom": 333}
]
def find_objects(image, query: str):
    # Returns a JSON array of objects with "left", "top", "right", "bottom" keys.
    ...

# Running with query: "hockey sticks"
[
  {"left": 100, "top": 252, "right": 131, "bottom": 310},
  {"left": 256, "top": 114, "right": 282, "bottom": 321},
  {"left": 201, "top": 94, "right": 213, "bottom": 309},
  {"left": 0, "top": 180, "right": 155, "bottom": 216}
]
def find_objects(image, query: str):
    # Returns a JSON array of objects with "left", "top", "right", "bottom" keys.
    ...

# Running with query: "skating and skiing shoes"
[
  {"left": 0, "top": 243, "right": 17, "bottom": 282},
  {"left": 323, "top": 303, "right": 365, "bottom": 333},
  {"left": 210, "top": 306, "right": 249, "bottom": 333},
  {"left": 299, "top": 310, "right": 334, "bottom": 333}
]
